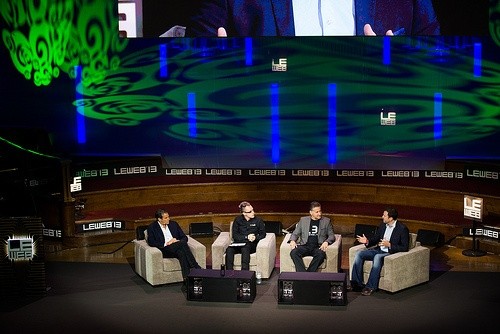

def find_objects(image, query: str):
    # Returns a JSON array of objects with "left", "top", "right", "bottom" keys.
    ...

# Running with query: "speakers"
[
  {"left": 354, "top": 224, "right": 379, "bottom": 246},
  {"left": 136, "top": 226, "right": 149, "bottom": 240},
  {"left": 416, "top": 229, "right": 445, "bottom": 248},
  {"left": 263, "top": 221, "right": 284, "bottom": 237},
  {"left": 189, "top": 222, "right": 214, "bottom": 238}
]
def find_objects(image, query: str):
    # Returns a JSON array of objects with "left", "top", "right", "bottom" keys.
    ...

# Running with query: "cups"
[{"left": 221, "top": 264, "right": 225, "bottom": 276}]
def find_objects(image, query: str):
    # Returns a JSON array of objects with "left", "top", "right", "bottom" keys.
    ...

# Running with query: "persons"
[
  {"left": 346, "top": 208, "right": 409, "bottom": 297},
  {"left": 186, "top": 0, "right": 442, "bottom": 36},
  {"left": 147, "top": 209, "right": 204, "bottom": 287},
  {"left": 225, "top": 201, "right": 267, "bottom": 271},
  {"left": 288, "top": 201, "right": 336, "bottom": 272}
]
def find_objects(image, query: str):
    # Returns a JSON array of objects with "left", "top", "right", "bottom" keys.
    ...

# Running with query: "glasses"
[{"left": 244, "top": 209, "right": 254, "bottom": 213}]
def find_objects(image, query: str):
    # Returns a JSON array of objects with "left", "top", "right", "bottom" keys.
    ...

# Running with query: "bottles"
[{"left": 256, "top": 270, "right": 262, "bottom": 285}]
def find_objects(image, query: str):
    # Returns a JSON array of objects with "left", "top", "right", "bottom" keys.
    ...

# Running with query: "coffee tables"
[
  {"left": 278, "top": 272, "right": 348, "bottom": 307},
  {"left": 187, "top": 268, "right": 257, "bottom": 304}
]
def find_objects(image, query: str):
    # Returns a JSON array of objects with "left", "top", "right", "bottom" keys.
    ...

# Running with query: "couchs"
[
  {"left": 212, "top": 222, "right": 276, "bottom": 279},
  {"left": 348, "top": 233, "right": 430, "bottom": 292},
  {"left": 279, "top": 233, "right": 341, "bottom": 273},
  {"left": 132, "top": 231, "right": 206, "bottom": 286}
]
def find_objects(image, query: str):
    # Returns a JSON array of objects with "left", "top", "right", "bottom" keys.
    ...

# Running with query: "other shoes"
[
  {"left": 346, "top": 286, "right": 361, "bottom": 292},
  {"left": 360, "top": 288, "right": 376, "bottom": 296}
]
